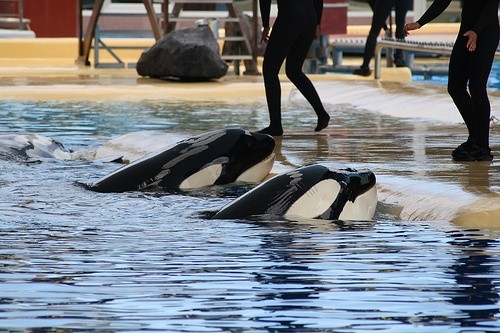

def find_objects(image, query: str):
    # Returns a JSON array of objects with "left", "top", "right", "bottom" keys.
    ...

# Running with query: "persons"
[
  {"left": 255, "top": 0, "right": 330, "bottom": 136},
  {"left": 401, "top": 0, "right": 500, "bottom": 161},
  {"left": 352, "top": 0, "right": 409, "bottom": 76}
]
